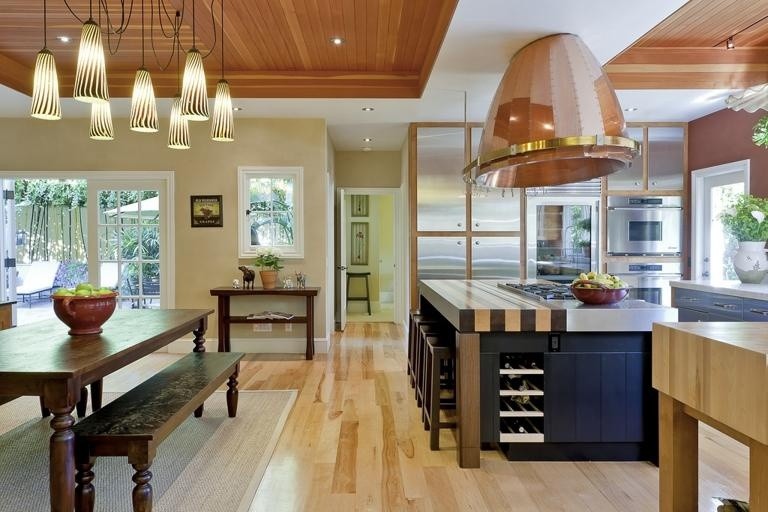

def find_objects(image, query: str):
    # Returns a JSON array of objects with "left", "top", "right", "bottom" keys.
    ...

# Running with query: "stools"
[
  {"left": 407, "top": 309, "right": 456, "bottom": 450},
  {"left": 346, "top": 272, "right": 371, "bottom": 315}
]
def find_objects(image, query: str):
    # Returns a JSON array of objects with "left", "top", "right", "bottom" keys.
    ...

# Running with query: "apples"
[{"left": 54, "top": 283, "right": 113, "bottom": 297}]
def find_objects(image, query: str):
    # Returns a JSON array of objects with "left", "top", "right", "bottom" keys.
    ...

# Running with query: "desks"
[
  {"left": 210, "top": 287, "right": 319, "bottom": 360},
  {"left": 0, "top": 308, "right": 215, "bottom": 512}
]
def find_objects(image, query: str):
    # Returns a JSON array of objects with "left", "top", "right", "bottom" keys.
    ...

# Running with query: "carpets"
[{"left": 0, "top": 389, "right": 300, "bottom": 512}]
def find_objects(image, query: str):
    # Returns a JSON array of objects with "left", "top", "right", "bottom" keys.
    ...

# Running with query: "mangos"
[{"left": 572, "top": 271, "right": 627, "bottom": 290}]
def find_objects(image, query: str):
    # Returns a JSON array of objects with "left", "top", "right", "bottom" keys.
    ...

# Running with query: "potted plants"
[
  {"left": 752, "top": 117, "right": 768, "bottom": 148},
  {"left": 255, "top": 254, "right": 285, "bottom": 289},
  {"left": 722, "top": 196, "right": 768, "bottom": 284}
]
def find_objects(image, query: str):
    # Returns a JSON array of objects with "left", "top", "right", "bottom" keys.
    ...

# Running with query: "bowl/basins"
[{"left": 51, "top": 293, "right": 117, "bottom": 335}]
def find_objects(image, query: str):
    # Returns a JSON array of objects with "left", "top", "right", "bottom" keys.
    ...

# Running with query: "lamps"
[{"left": 31, "top": 0, "right": 234, "bottom": 150}]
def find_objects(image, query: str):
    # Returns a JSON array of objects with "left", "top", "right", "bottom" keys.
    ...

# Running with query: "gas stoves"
[{"left": 499, "top": 283, "right": 575, "bottom": 300}]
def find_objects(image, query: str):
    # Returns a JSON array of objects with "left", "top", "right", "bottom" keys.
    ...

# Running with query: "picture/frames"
[
  {"left": 238, "top": 167, "right": 304, "bottom": 259},
  {"left": 350, "top": 222, "right": 369, "bottom": 265},
  {"left": 351, "top": 195, "right": 369, "bottom": 217},
  {"left": 190, "top": 195, "right": 223, "bottom": 227}
]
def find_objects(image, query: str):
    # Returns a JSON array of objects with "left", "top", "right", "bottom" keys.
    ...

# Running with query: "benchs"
[{"left": 74, "top": 352, "right": 247, "bottom": 512}]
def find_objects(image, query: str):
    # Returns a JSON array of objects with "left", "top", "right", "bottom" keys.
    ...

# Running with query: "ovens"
[
  {"left": 607, "top": 195, "right": 683, "bottom": 258},
  {"left": 606, "top": 262, "right": 684, "bottom": 307}
]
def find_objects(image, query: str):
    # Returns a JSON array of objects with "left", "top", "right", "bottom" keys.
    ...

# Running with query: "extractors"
[{"left": 461, "top": 33, "right": 640, "bottom": 189}]
